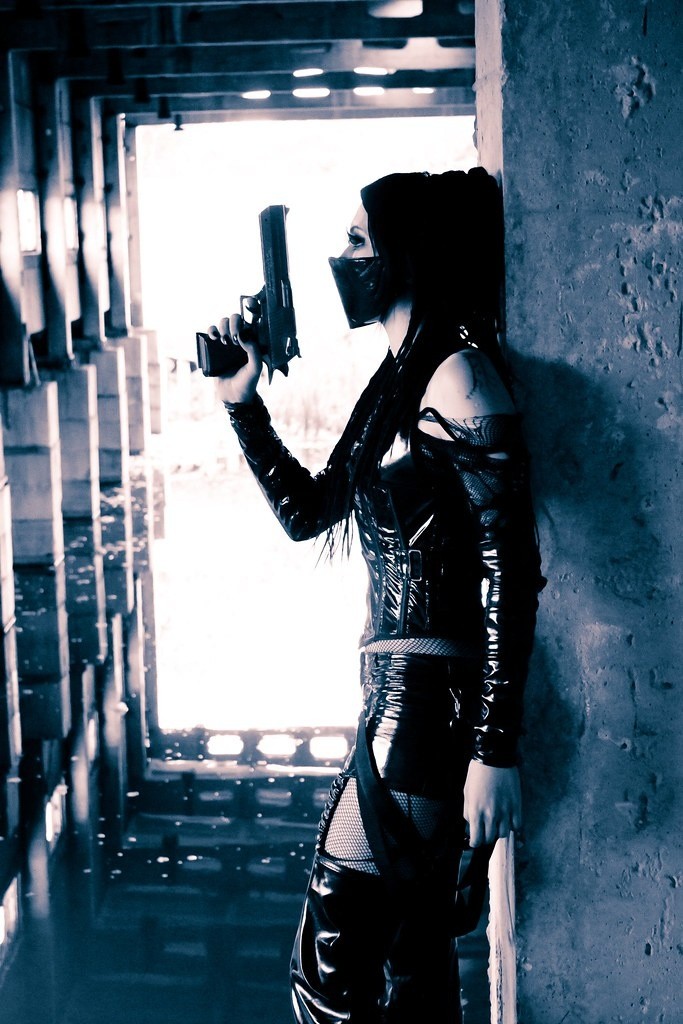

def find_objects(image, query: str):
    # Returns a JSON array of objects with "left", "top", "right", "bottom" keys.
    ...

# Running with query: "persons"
[{"left": 206, "top": 165, "right": 539, "bottom": 1024}]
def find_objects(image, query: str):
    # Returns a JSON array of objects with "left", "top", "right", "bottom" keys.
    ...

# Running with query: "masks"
[{"left": 326, "top": 256, "right": 405, "bottom": 331}]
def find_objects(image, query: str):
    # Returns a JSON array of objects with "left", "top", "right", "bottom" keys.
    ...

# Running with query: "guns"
[{"left": 196, "top": 204, "right": 304, "bottom": 386}]
